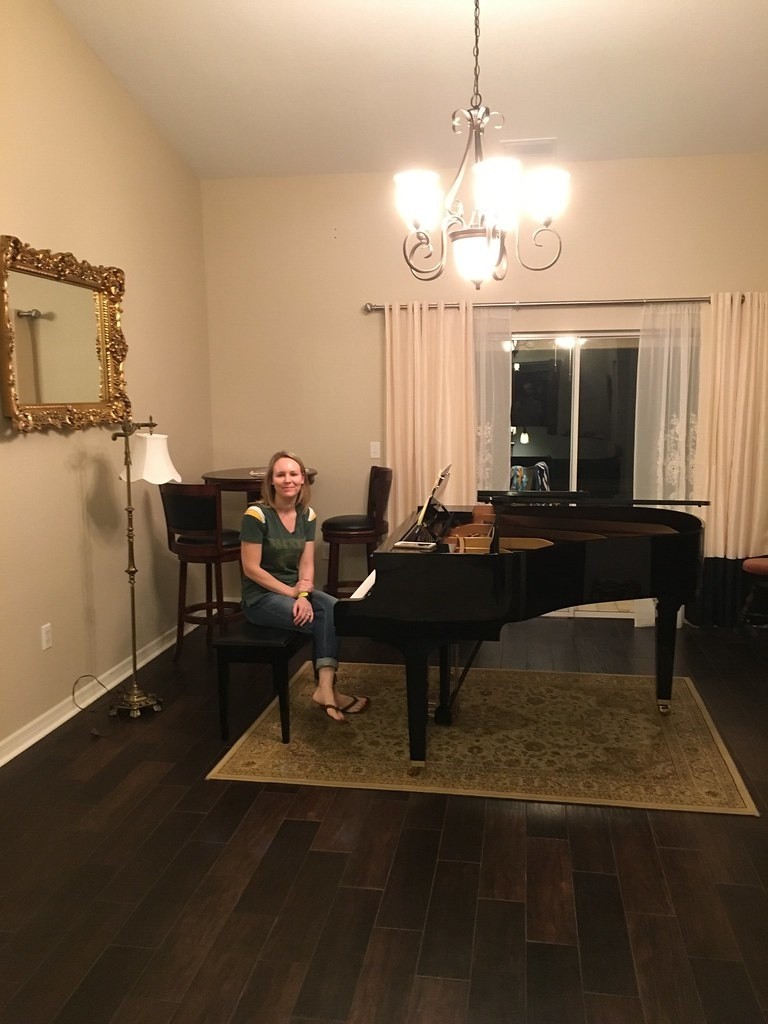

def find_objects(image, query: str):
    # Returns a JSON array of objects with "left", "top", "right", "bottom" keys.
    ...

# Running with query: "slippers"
[
  {"left": 321, "top": 705, "right": 345, "bottom": 722},
  {"left": 341, "top": 695, "right": 371, "bottom": 714}
]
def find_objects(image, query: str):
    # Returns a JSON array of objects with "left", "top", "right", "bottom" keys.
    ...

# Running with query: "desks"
[{"left": 200, "top": 467, "right": 318, "bottom": 508}]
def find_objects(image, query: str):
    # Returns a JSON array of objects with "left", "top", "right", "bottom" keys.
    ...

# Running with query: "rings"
[{"left": 306, "top": 613, "right": 311, "bottom": 616}]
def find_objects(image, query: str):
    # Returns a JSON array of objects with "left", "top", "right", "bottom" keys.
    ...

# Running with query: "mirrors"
[{"left": 0, "top": 235, "right": 133, "bottom": 432}]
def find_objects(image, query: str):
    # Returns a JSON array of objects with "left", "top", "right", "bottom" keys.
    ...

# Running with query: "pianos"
[{"left": 334, "top": 490, "right": 713, "bottom": 760}]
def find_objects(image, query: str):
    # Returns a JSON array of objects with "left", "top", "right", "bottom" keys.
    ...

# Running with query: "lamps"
[
  {"left": 109, "top": 412, "right": 182, "bottom": 717},
  {"left": 392, "top": 0, "right": 572, "bottom": 290}
]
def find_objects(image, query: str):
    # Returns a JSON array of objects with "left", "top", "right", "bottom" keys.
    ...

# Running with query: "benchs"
[{"left": 212, "top": 621, "right": 321, "bottom": 744}]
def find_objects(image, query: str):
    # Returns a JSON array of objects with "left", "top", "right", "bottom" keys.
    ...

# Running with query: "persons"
[{"left": 239, "top": 449, "right": 370, "bottom": 725}]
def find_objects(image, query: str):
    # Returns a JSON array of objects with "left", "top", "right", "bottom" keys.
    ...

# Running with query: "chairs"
[
  {"left": 159, "top": 482, "right": 245, "bottom": 658},
  {"left": 322, "top": 466, "right": 393, "bottom": 599},
  {"left": 510, "top": 465, "right": 538, "bottom": 491},
  {"left": 731, "top": 558, "right": 768, "bottom": 645}
]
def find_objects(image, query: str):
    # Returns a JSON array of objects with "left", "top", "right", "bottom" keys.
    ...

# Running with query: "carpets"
[{"left": 205, "top": 660, "right": 761, "bottom": 817}]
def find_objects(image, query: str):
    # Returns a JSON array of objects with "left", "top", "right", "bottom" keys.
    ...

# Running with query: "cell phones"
[{"left": 395, "top": 541, "right": 436, "bottom": 550}]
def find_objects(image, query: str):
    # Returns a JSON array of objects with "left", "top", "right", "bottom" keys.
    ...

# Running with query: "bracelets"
[{"left": 297, "top": 591, "right": 313, "bottom": 603}]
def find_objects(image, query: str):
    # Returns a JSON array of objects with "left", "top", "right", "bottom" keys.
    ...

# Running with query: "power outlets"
[{"left": 41, "top": 623, "right": 52, "bottom": 650}]
